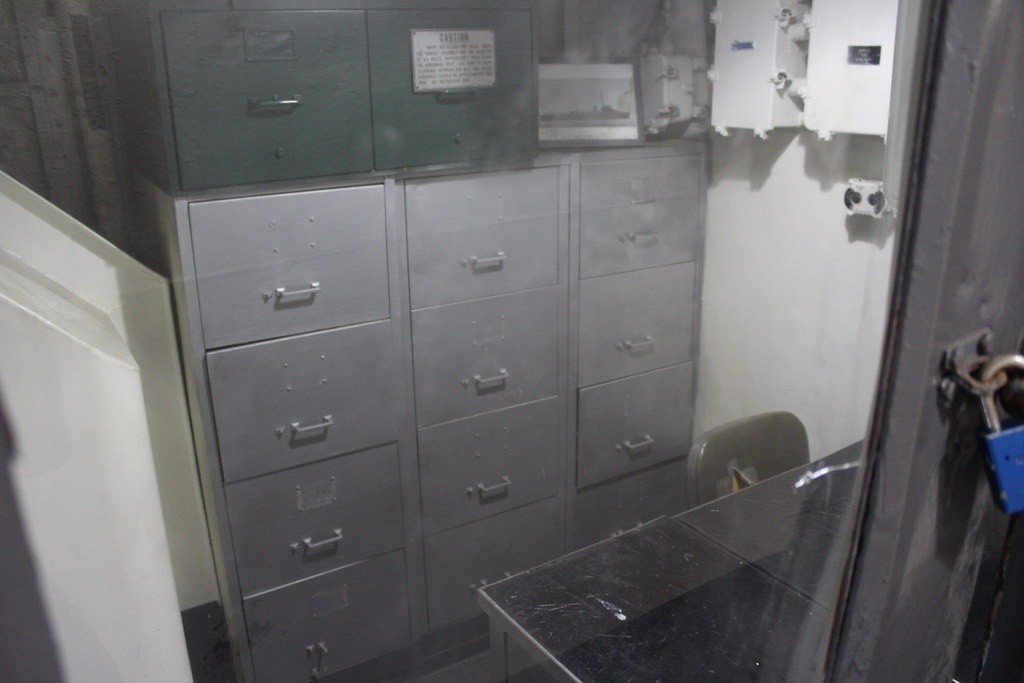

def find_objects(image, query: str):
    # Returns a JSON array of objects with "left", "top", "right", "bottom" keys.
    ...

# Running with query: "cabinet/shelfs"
[{"left": 92, "top": 0, "right": 711, "bottom": 683}]
